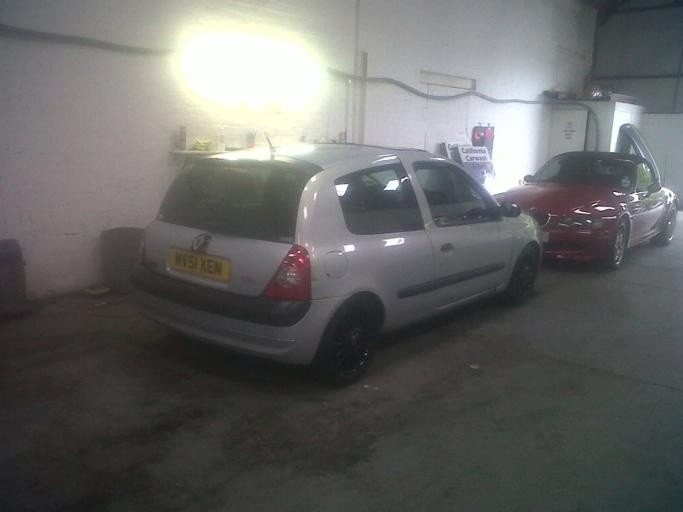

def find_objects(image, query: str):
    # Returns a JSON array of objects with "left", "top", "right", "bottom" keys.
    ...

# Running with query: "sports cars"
[{"left": 491, "top": 148, "right": 679, "bottom": 274}]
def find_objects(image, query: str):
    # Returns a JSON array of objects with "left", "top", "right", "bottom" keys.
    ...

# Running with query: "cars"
[{"left": 127, "top": 138, "right": 545, "bottom": 384}]
